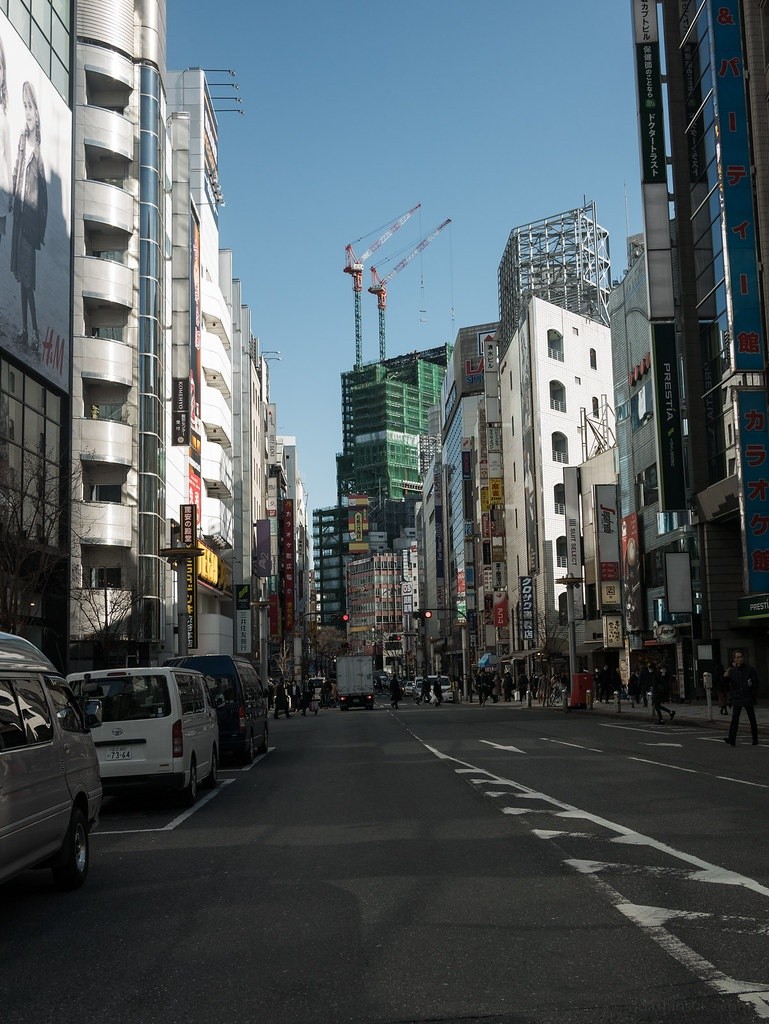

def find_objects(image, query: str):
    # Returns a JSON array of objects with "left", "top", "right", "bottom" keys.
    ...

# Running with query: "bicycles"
[
  {"left": 478, "top": 683, "right": 491, "bottom": 706},
  {"left": 551, "top": 688, "right": 566, "bottom": 707}
]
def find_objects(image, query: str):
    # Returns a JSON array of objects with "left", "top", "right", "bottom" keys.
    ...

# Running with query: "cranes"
[
  {"left": 366, "top": 218, "right": 456, "bottom": 360},
  {"left": 341, "top": 201, "right": 423, "bottom": 368}
]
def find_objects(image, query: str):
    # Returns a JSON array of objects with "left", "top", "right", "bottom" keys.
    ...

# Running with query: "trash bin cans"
[{"left": 572, "top": 673, "right": 593, "bottom": 709}]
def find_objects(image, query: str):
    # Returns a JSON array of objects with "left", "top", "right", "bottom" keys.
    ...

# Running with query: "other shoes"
[
  {"left": 720, "top": 710, "right": 723, "bottom": 714},
  {"left": 391, "top": 703, "right": 394, "bottom": 709},
  {"left": 752, "top": 740, "right": 758, "bottom": 745},
  {"left": 301, "top": 713, "right": 306, "bottom": 716},
  {"left": 723, "top": 712, "right": 728, "bottom": 715},
  {"left": 315, "top": 713, "right": 319, "bottom": 716},
  {"left": 395, "top": 707, "right": 399, "bottom": 709},
  {"left": 286, "top": 715, "right": 293, "bottom": 719},
  {"left": 273, "top": 716, "right": 280, "bottom": 719},
  {"left": 670, "top": 711, "right": 675, "bottom": 720},
  {"left": 654, "top": 721, "right": 664, "bottom": 725},
  {"left": 416, "top": 702, "right": 419, "bottom": 705},
  {"left": 724, "top": 738, "right": 735, "bottom": 746}
]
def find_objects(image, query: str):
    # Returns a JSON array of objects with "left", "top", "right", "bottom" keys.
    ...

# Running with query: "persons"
[
  {"left": 447, "top": 673, "right": 464, "bottom": 696},
  {"left": 268, "top": 674, "right": 337, "bottom": 720},
  {"left": 627, "top": 664, "right": 676, "bottom": 725},
  {"left": 433, "top": 676, "right": 443, "bottom": 707},
  {"left": 517, "top": 671, "right": 528, "bottom": 703},
  {"left": 593, "top": 664, "right": 622, "bottom": 704},
  {"left": 467, "top": 670, "right": 515, "bottom": 703},
  {"left": 416, "top": 676, "right": 432, "bottom": 706},
  {"left": 529, "top": 673, "right": 567, "bottom": 705},
  {"left": 389, "top": 674, "right": 403, "bottom": 709},
  {"left": 715, "top": 665, "right": 729, "bottom": 715},
  {"left": 724, "top": 652, "right": 760, "bottom": 746},
  {"left": 0, "top": 36, "right": 48, "bottom": 349}
]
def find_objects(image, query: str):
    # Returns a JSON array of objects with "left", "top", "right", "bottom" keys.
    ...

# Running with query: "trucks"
[{"left": 334, "top": 654, "right": 377, "bottom": 711}]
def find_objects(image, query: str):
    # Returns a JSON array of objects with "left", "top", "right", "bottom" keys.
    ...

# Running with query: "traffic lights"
[
  {"left": 330, "top": 613, "right": 351, "bottom": 623},
  {"left": 388, "top": 635, "right": 401, "bottom": 641},
  {"left": 411, "top": 611, "right": 432, "bottom": 619}
]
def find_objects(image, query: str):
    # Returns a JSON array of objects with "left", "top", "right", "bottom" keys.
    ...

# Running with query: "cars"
[{"left": 374, "top": 675, "right": 454, "bottom": 703}]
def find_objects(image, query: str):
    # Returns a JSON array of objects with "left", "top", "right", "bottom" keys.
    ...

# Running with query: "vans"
[
  {"left": 65, "top": 665, "right": 234, "bottom": 805},
  {"left": 0, "top": 628, "right": 107, "bottom": 897},
  {"left": 306, "top": 676, "right": 327, "bottom": 700},
  {"left": 162, "top": 653, "right": 273, "bottom": 768}
]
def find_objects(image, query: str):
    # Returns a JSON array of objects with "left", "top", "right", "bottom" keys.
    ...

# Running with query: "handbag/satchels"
[
  {"left": 514, "top": 690, "right": 521, "bottom": 701},
  {"left": 310, "top": 702, "right": 319, "bottom": 712},
  {"left": 429, "top": 694, "right": 438, "bottom": 704},
  {"left": 536, "top": 691, "right": 538, "bottom": 697}
]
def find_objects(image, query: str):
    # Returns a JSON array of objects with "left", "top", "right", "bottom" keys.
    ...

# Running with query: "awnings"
[
  {"left": 478, "top": 650, "right": 541, "bottom": 667},
  {"left": 563, "top": 643, "right": 604, "bottom": 655}
]
